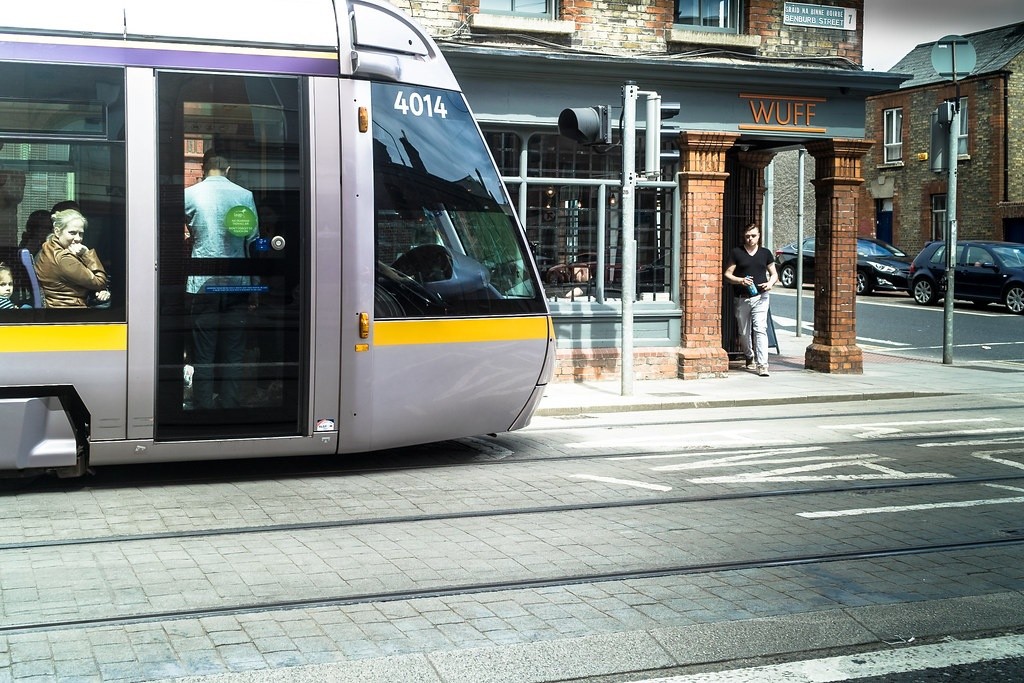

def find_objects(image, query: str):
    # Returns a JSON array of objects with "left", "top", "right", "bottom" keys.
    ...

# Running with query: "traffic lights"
[
  {"left": 647, "top": 94, "right": 681, "bottom": 179},
  {"left": 558, "top": 103, "right": 612, "bottom": 148}
]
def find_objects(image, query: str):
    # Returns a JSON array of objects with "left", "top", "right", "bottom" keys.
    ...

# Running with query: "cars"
[
  {"left": 546, "top": 254, "right": 595, "bottom": 286},
  {"left": 907, "top": 238, "right": 1024, "bottom": 315},
  {"left": 640, "top": 254, "right": 669, "bottom": 286},
  {"left": 774, "top": 233, "right": 915, "bottom": 296}
]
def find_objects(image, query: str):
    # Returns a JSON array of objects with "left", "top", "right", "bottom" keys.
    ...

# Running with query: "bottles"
[{"left": 745, "top": 276, "right": 758, "bottom": 295}]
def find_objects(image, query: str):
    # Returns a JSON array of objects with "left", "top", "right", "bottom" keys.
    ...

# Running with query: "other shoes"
[
  {"left": 746, "top": 357, "right": 757, "bottom": 370},
  {"left": 759, "top": 366, "right": 769, "bottom": 376}
]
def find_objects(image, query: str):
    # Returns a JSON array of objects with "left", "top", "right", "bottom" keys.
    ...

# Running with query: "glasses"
[{"left": 744, "top": 234, "right": 758, "bottom": 238}]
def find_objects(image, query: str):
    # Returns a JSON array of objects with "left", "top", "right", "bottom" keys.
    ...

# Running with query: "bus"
[{"left": 1, "top": 0, "right": 560, "bottom": 477}]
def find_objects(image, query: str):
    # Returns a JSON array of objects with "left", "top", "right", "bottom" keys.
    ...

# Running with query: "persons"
[
  {"left": 1, "top": 260, "right": 36, "bottom": 309},
  {"left": 724, "top": 225, "right": 779, "bottom": 377},
  {"left": 33, "top": 209, "right": 112, "bottom": 306},
  {"left": 183, "top": 148, "right": 261, "bottom": 409},
  {"left": 18, "top": 210, "right": 52, "bottom": 259}
]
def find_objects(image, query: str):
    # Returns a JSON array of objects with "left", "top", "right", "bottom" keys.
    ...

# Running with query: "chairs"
[{"left": 17, "top": 248, "right": 42, "bottom": 308}]
